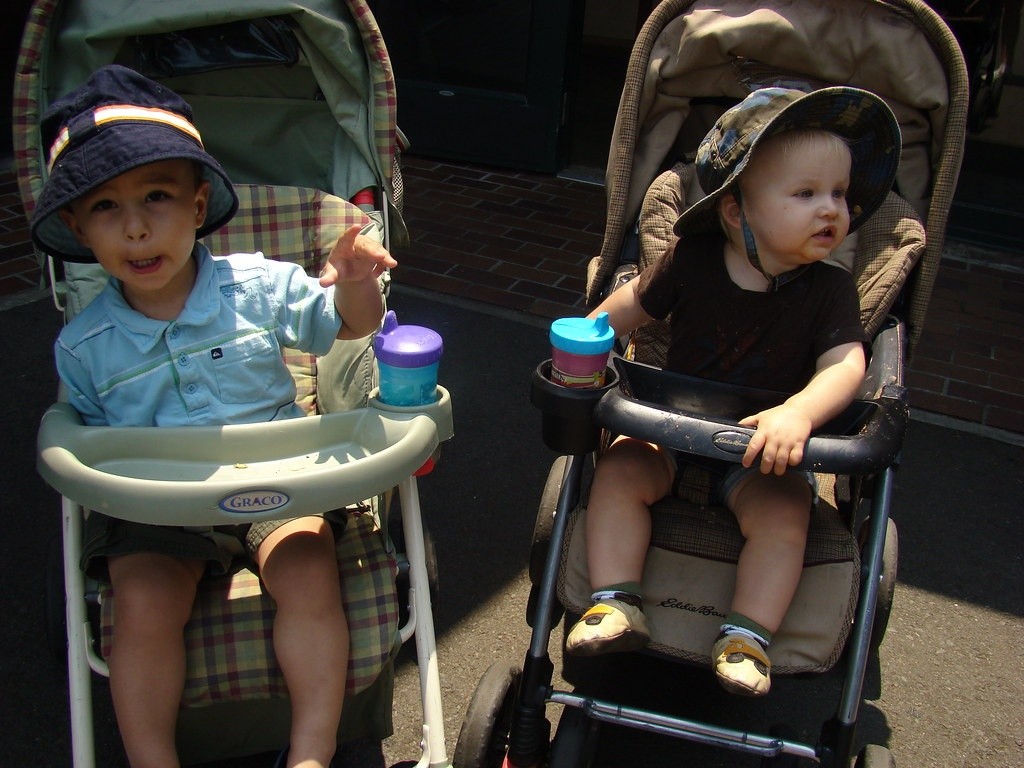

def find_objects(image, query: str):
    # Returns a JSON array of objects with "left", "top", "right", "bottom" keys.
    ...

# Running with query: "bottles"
[
  {"left": 373, "top": 310, "right": 442, "bottom": 406},
  {"left": 549, "top": 312, "right": 614, "bottom": 388}
]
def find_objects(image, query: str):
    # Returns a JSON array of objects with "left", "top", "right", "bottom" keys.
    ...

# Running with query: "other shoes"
[
  {"left": 566, "top": 591, "right": 652, "bottom": 658},
  {"left": 710, "top": 630, "right": 772, "bottom": 698}
]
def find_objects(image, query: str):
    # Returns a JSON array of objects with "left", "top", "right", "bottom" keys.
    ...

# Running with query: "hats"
[
  {"left": 30, "top": 64, "right": 239, "bottom": 263},
  {"left": 672, "top": 87, "right": 903, "bottom": 248}
]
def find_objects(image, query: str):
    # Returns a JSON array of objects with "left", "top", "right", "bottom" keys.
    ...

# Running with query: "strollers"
[
  {"left": 448, "top": 2, "right": 974, "bottom": 768},
  {"left": 0, "top": 0, "right": 455, "bottom": 768}
]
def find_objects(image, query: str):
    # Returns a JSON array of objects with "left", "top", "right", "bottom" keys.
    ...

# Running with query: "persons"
[
  {"left": 565, "top": 87, "right": 902, "bottom": 698},
  {"left": 28, "top": 65, "right": 397, "bottom": 768}
]
